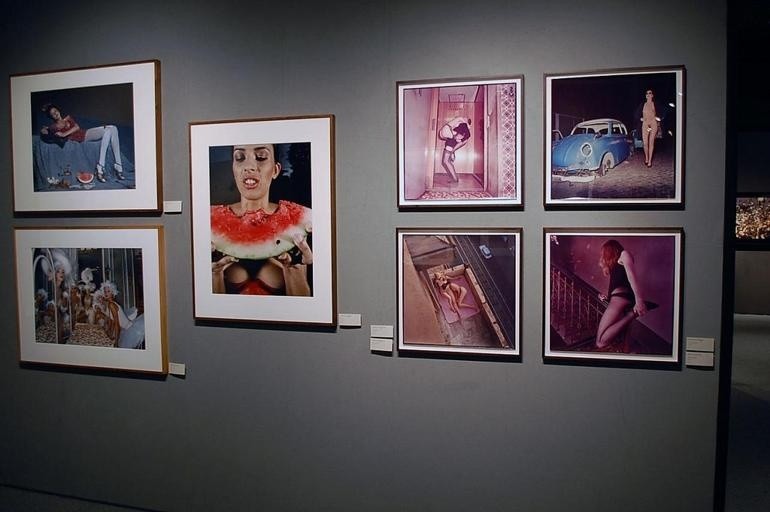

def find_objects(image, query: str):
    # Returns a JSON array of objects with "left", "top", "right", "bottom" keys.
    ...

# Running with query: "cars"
[
  {"left": 552, "top": 118, "right": 635, "bottom": 176},
  {"left": 629, "top": 129, "right": 643, "bottom": 149}
]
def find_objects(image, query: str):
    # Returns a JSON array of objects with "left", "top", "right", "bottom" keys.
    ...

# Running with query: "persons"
[
  {"left": 641, "top": 90, "right": 657, "bottom": 167},
  {"left": 41, "top": 106, "right": 126, "bottom": 183},
  {"left": 596, "top": 238, "right": 661, "bottom": 350},
  {"left": 35, "top": 249, "right": 132, "bottom": 348},
  {"left": 434, "top": 272, "right": 480, "bottom": 319},
  {"left": 439, "top": 116, "right": 472, "bottom": 184},
  {"left": 210, "top": 143, "right": 313, "bottom": 294}
]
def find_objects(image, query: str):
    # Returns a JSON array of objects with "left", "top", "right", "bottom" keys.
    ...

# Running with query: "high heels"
[
  {"left": 623, "top": 300, "right": 661, "bottom": 314},
  {"left": 113, "top": 162, "right": 126, "bottom": 180},
  {"left": 93, "top": 162, "right": 106, "bottom": 184}
]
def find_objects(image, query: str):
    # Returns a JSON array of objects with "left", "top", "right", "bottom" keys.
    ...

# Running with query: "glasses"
[{"left": 645, "top": 92, "right": 653, "bottom": 96}]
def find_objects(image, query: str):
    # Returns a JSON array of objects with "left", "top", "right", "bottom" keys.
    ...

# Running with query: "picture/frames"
[
  {"left": 188, "top": 113, "right": 338, "bottom": 333},
  {"left": 395, "top": 225, "right": 523, "bottom": 362},
  {"left": 11, "top": 224, "right": 169, "bottom": 378},
  {"left": 396, "top": 74, "right": 526, "bottom": 212},
  {"left": 542, "top": 226, "right": 686, "bottom": 372},
  {"left": 8, "top": 59, "right": 163, "bottom": 218},
  {"left": 543, "top": 64, "right": 688, "bottom": 212}
]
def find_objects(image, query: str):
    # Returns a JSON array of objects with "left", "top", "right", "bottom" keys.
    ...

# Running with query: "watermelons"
[
  {"left": 75, "top": 174, "right": 94, "bottom": 183},
  {"left": 211, "top": 200, "right": 314, "bottom": 259}
]
